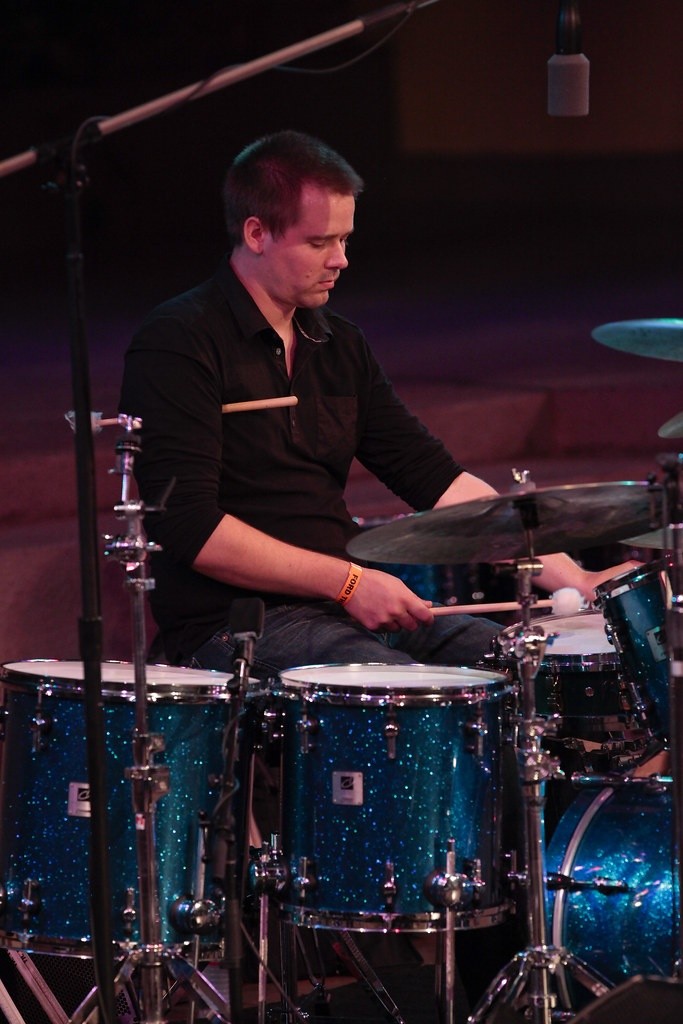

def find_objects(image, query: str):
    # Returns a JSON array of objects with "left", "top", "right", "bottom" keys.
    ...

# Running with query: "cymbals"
[
  {"left": 658, "top": 409, "right": 683, "bottom": 439},
  {"left": 591, "top": 317, "right": 683, "bottom": 360},
  {"left": 345, "top": 483, "right": 671, "bottom": 566}
]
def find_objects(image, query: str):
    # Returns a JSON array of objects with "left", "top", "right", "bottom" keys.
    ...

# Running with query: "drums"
[
  {"left": 496, "top": 606, "right": 648, "bottom": 751},
  {"left": 1, "top": 657, "right": 263, "bottom": 957},
  {"left": 592, "top": 554, "right": 683, "bottom": 746},
  {"left": 271, "top": 662, "right": 510, "bottom": 929},
  {"left": 540, "top": 784, "right": 681, "bottom": 981}
]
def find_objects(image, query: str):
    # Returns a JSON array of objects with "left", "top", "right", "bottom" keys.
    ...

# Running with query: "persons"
[{"left": 119, "top": 129, "right": 645, "bottom": 782}]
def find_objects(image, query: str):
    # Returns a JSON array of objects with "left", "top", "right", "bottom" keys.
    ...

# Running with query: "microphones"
[
  {"left": 547, "top": 1, "right": 590, "bottom": 116},
  {"left": 228, "top": 596, "right": 264, "bottom": 694}
]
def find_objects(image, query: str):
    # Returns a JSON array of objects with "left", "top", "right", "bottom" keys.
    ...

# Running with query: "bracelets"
[{"left": 334, "top": 562, "right": 362, "bottom": 607}]
